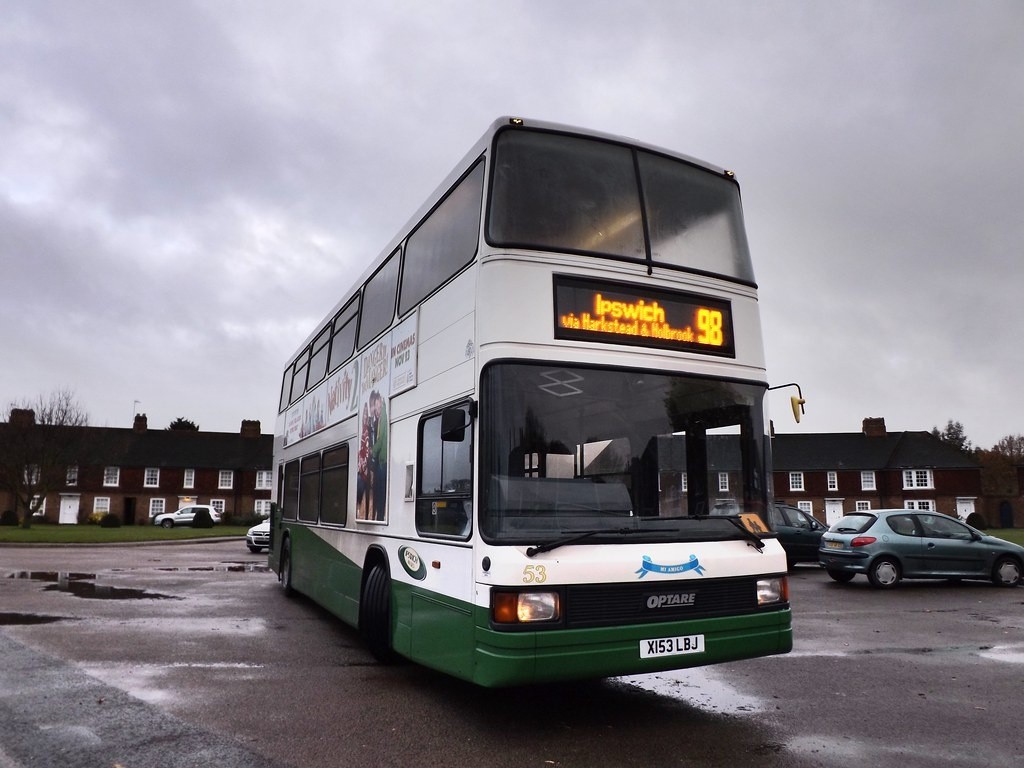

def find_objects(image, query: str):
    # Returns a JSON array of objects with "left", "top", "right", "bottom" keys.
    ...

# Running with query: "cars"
[
  {"left": 819, "top": 497, "right": 1024, "bottom": 588},
  {"left": 247, "top": 516, "right": 271, "bottom": 552}
]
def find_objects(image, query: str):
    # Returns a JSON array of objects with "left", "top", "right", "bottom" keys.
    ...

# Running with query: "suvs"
[
  {"left": 709, "top": 500, "right": 829, "bottom": 572},
  {"left": 153, "top": 504, "right": 222, "bottom": 529}
]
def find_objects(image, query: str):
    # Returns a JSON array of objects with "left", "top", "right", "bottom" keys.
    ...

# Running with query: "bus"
[{"left": 267, "top": 113, "right": 806, "bottom": 693}]
